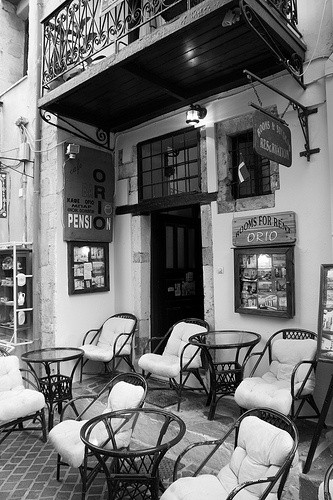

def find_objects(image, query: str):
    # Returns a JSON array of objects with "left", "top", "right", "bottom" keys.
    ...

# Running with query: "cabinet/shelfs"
[{"left": 0, "top": 243, "right": 33, "bottom": 346}]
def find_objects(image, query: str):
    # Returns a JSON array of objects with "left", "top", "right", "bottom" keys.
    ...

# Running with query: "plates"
[{"left": 2, "top": 256, "right": 13, "bottom": 269}]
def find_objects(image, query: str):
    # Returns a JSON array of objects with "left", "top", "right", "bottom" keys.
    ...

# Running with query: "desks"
[
  {"left": 80, "top": 408, "right": 187, "bottom": 500},
  {"left": 188, "top": 327, "right": 262, "bottom": 420},
  {"left": 21, "top": 347, "right": 85, "bottom": 433}
]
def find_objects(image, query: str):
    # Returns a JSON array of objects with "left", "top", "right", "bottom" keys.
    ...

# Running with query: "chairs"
[
  {"left": 79, "top": 313, "right": 138, "bottom": 386},
  {"left": 0, "top": 346, "right": 48, "bottom": 443},
  {"left": 49, "top": 372, "right": 148, "bottom": 500},
  {"left": 138, "top": 318, "right": 210, "bottom": 412},
  {"left": 234, "top": 328, "right": 328, "bottom": 431},
  {"left": 159, "top": 406, "right": 299, "bottom": 500}
]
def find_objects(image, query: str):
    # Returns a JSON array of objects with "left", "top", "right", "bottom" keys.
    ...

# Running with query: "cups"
[{"left": 0, "top": 263, "right": 22, "bottom": 305}]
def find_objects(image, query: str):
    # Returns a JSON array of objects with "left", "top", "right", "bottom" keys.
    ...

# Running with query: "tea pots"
[{"left": 17, "top": 291, "right": 25, "bottom": 305}]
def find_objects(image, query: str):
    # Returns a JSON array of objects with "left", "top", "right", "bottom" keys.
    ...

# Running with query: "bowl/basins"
[{"left": 5, "top": 301, "right": 14, "bottom": 305}]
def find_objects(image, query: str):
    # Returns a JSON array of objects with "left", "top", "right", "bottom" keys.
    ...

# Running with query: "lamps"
[{"left": 186, "top": 102, "right": 207, "bottom": 126}]
